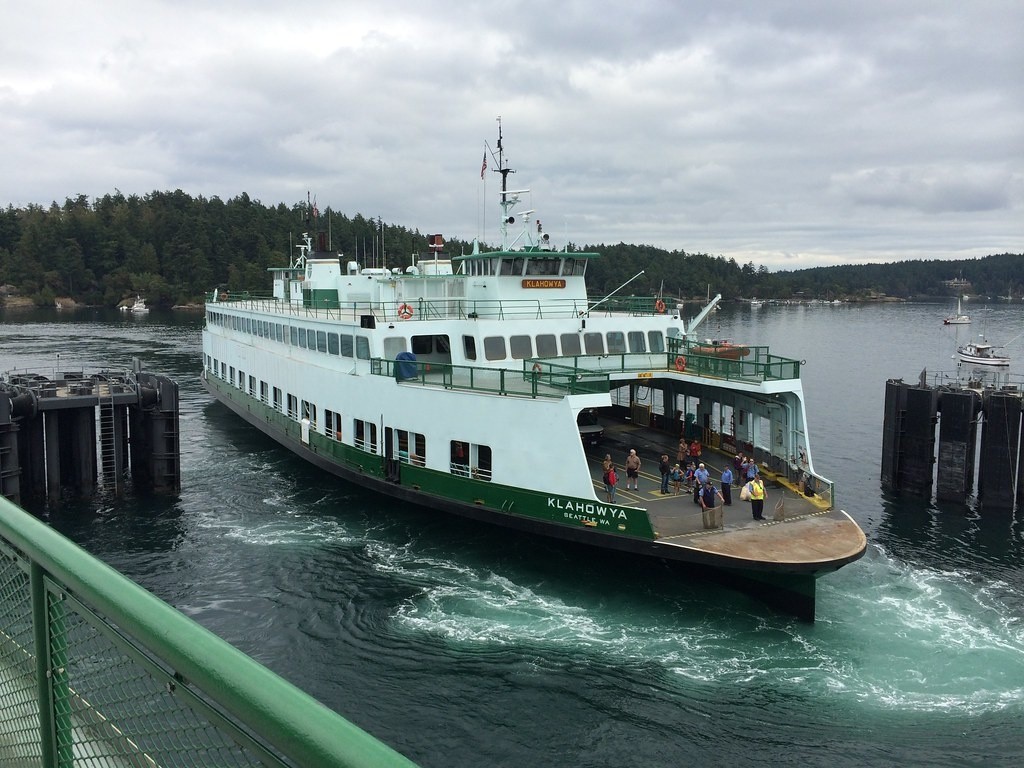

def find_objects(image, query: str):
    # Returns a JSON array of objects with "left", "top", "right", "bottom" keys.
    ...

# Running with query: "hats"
[
  {"left": 707, "top": 480, "right": 713, "bottom": 485},
  {"left": 723, "top": 464, "right": 730, "bottom": 467}
]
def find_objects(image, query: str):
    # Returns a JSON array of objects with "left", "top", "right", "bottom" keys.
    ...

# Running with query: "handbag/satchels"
[
  {"left": 680, "top": 478, "right": 684, "bottom": 482},
  {"left": 697, "top": 450, "right": 701, "bottom": 456}
]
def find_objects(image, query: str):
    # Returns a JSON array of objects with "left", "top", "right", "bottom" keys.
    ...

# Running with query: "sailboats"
[
  {"left": 943, "top": 296, "right": 972, "bottom": 325},
  {"left": 956, "top": 307, "right": 1011, "bottom": 366},
  {"left": 701, "top": 283, "right": 717, "bottom": 313}
]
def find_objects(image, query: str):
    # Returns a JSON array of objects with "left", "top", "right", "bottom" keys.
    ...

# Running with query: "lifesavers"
[
  {"left": 675, "top": 356, "right": 686, "bottom": 370},
  {"left": 532, "top": 363, "right": 542, "bottom": 379},
  {"left": 398, "top": 304, "right": 414, "bottom": 319},
  {"left": 655, "top": 300, "right": 666, "bottom": 313}
]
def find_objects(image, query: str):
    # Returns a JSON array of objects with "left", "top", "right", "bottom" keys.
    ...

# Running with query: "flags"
[
  {"left": 481, "top": 152, "right": 487, "bottom": 179},
  {"left": 313, "top": 201, "right": 317, "bottom": 216}
]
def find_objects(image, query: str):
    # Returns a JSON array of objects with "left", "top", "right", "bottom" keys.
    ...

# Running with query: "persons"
[
  {"left": 659, "top": 455, "right": 671, "bottom": 494},
  {"left": 626, "top": 450, "right": 640, "bottom": 491},
  {"left": 734, "top": 452, "right": 760, "bottom": 484},
  {"left": 677, "top": 439, "right": 688, "bottom": 471},
  {"left": 603, "top": 454, "right": 619, "bottom": 504},
  {"left": 671, "top": 464, "right": 684, "bottom": 495},
  {"left": 694, "top": 463, "right": 710, "bottom": 504},
  {"left": 722, "top": 465, "right": 733, "bottom": 505},
  {"left": 699, "top": 480, "right": 725, "bottom": 528},
  {"left": 691, "top": 439, "right": 700, "bottom": 469},
  {"left": 685, "top": 465, "right": 692, "bottom": 493},
  {"left": 749, "top": 474, "right": 768, "bottom": 521}
]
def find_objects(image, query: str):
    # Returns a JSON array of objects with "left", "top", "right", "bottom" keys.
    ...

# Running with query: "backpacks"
[{"left": 603, "top": 471, "right": 611, "bottom": 485}]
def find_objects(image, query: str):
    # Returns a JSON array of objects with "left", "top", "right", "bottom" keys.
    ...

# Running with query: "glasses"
[{"left": 750, "top": 461, "right": 753, "bottom": 462}]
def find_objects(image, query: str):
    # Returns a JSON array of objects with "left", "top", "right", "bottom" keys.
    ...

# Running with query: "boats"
[
  {"left": 131, "top": 307, "right": 150, "bottom": 313},
  {"left": 749, "top": 297, "right": 841, "bottom": 304},
  {"left": 200, "top": 110, "right": 868, "bottom": 628}
]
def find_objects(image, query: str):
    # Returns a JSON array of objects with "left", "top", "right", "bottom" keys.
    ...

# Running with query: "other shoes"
[
  {"left": 609, "top": 501, "right": 616, "bottom": 504},
  {"left": 666, "top": 491, "right": 670, "bottom": 493},
  {"left": 674, "top": 491, "right": 680, "bottom": 496},
  {"left": 753, "top": 517, "right": 760, "bottom": 521},
  {"left": 724, "top": 502, "right": 732, "bottom": 506},
  {"left": 635, "top": 488, "right": 639, "bottom": 491},
  {"left": 661, "top": 491, "right": 665, "bottom": 494},
  {"left": 626, "top": 488, "right": 629, "bottom": 491},
  {"left": 760, "top": 517, "right": 766, "bottom": 520},
  {"left": 685, "top": 490, "right": 692, "bottom": 493}
]
